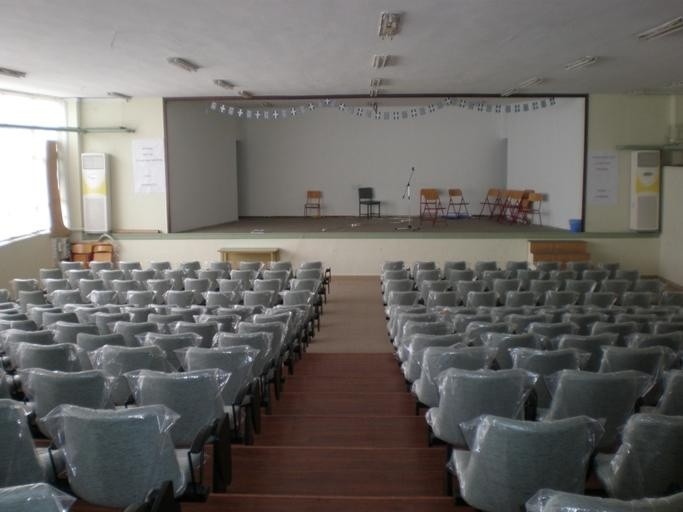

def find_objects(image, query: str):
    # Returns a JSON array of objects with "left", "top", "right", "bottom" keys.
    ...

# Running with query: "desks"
[{"left": 217, "top": 248, "right": 278, "bottom": 270}]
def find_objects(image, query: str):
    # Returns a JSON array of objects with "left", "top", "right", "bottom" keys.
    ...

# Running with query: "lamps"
[
  {"left": 0, "top": 67, "right": 26, "bottom": 77},
  {"left": 370, "top": 78, "right": 382, "bottom": 98},
  {"left": 562, "top": 55, "right": 600, "bottom": 72},
  {"left": 519, "top": 77, "right": 544, "bottom": 88},
  {"left": 214, "top": 79, "right": 234, "bottom": 90},
  {"left": 379, "top": 12, "right": 401, "bottom": 40},
  {"left": 634, "top": 16, "right": 683, "bottom": 42},
  {"left": 168, "top": 58, "right": 200, "bottom": 73},
  {"left": 372, "top": 55, "right": 391, "bottom": 69}
]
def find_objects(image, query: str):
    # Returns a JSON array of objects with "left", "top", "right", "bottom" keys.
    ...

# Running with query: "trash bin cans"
[{"left": 569, "top": 219, "right": 583, "bottom": 233}]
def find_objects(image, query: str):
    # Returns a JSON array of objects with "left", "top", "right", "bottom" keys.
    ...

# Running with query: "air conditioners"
[
  {"left": 81, "top": 153, "right": 110, "bottom": 235},
  {"left": 630, "top": 150, "right": 660, "bottom": 231}
]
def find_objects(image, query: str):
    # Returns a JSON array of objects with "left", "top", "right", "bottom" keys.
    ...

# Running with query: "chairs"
[
  {"left": 418, "top": 189, "right": 449, "bottom": 228},
  {"left": 445, "top": 189, "right": 470, "bottom": 222},
  {"left": 304, "top": 190, "right": 322, "bottom": 218},
  {"left": 359, "top": 188, "right": 381, "bottom": 219},
  {"left": 71, "top": 243, "right": 113, "bottom": 262},
  {"left": 478, "top": 188, "right": 543, "bottom": 226}
]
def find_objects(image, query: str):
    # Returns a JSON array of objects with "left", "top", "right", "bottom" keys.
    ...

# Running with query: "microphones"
[{"left": 412, "top": 167, "right": 415, "bottom": 171}]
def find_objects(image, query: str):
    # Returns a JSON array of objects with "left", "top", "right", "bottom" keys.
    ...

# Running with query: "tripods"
[{"left": 395, "top": 171, "right": 423, "bottom": 232}]
